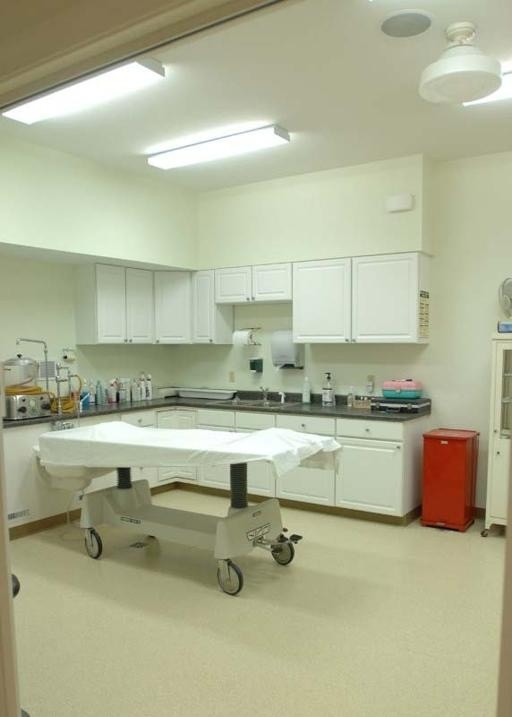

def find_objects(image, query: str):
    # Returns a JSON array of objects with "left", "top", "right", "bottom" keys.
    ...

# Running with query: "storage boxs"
[
  {"left": 382, "top": 380, "right": 425, "bottom": 399},
  {"left": 370, "top": 397, "right": 431, "bottom": 416}
]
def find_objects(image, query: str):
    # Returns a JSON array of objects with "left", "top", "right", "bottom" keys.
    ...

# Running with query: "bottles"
[
  {"left": 108, "top": 371, "right": 153, "bottom": 402},
  {"left": 347, "top": 385, "right": 355, "bottom": 408},
  {"left": 87, "top": 377, "right": 96, "bottom": 405},
  {"left": 81, "top": 378, "right": 89, "bottom": 410},
  {"left": 96, "top": 379, "right": 104, "bottom": 405}
]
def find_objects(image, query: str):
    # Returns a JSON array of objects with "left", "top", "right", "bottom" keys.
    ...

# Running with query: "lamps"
[
  {"left": 1, "top": 54, "right": 167, "bottom": 127},
  {"left": 147, "top": 120, "right": 292, "bottom": 170}
]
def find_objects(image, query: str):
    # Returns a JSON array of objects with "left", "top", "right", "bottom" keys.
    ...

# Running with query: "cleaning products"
[{"left": 347, "top": 386, "right": 354, "bottom": 406}]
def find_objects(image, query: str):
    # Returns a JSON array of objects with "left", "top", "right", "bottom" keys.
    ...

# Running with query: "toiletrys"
[{"left": 322, "top": 372, "right": 336, "bottom": 406}]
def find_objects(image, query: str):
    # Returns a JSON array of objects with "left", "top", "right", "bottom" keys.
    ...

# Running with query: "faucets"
[{"left": 260, "top": 386, "right": 271, "bottom": 402}]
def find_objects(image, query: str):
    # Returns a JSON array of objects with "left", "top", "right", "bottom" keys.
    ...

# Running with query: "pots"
[{"left": 3, "top": 351, "right": 40, "bottom": 387}]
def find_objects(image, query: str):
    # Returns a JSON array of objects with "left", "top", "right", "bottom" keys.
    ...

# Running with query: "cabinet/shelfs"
[
  {"left": 154, "top": 267, "right": 191, "bottom": 347},
  {"left": 191, "top": 268, "right": 236, "bottom": 346},
  {"left": 334, "top": 409, "right": 431, "bottom": 529},
  {"left": 73, "top": 262, "right": 154, "bottom": 347},
  {"left": 121, "top": 407, "right": 155, "bottom": 495},
  {"left": 214, "top": 261, "right": 292, "bottom": 305},
  {"left": 155, "top": 402, "right": 197, "bottom": 495},
  {"left": 195, "top": 407, "right": 275, "bottom": 502},
  {"left": 477, "top": 328, "right": 511, "bottom": 537},
  {"left": 276, "top": 408, "right": 335, "bottom": 508},
  {"left": 291, "top": 251, "right": 431, "bottom": 346}
]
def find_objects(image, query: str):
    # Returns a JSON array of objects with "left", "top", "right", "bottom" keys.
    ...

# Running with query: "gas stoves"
[{"left": 1, "top": 392, "right": 54, "bottom": 419}]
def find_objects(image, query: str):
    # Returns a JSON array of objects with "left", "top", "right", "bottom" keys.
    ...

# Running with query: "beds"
[{"left": 39, "top": 419, "right": 335, "bottom": 597}]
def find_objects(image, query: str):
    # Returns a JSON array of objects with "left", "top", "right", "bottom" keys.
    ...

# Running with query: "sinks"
[
  {"left": 207, "top": 400, "right": 252, "bottom": 407},
  {"left": 252, "top": 401, "right": 298, "bottom": 410}
]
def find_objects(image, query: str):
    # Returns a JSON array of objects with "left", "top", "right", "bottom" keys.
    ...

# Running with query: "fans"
[
  {"left": 416, "top": 18, "right": 502, "bottom": 105},
  {"left": 494, "top": 273, "right": 512, "bottom": 321}
]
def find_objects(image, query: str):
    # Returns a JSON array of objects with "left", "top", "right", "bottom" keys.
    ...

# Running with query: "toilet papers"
[
  {"left": 63, "top": 354, "right": 75, "bottom": 362},
  {"left": 231, "top": 330, "right": 255, "bottom": 347}
]
